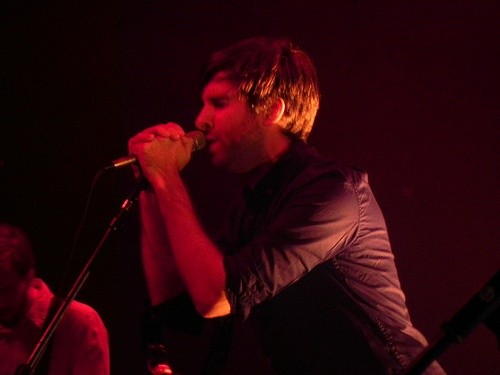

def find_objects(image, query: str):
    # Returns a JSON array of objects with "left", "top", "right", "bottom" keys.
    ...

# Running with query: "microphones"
[{"left": 111, "top": 131, "right": 206, "bottom": 169}]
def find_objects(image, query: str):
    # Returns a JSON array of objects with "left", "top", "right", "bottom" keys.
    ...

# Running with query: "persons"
[
  {"left": 0, "top": 224, "right": 112, "bottom": 375},
  {"left": 126, "top": 33, "right": 446, "bottom": 375}
]
